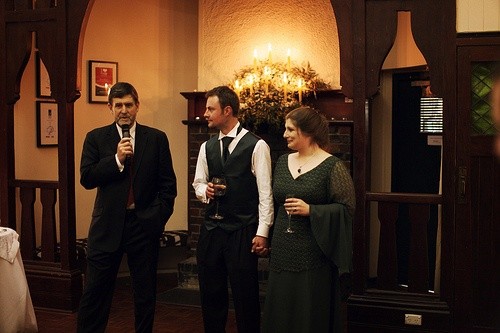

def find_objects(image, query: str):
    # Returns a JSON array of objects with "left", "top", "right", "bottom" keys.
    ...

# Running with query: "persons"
[
  {"left": 75, "top": 82, "right": 178, "bottom": 333},
  {"left": 191, "top": 86, "right": 275, "bottom": 333},
  {"left": 255, "top": 108, "right": 356, "bottom": 333}
]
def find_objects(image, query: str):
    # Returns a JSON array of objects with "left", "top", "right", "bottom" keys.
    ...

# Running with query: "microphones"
[{"left": 122, "top": 124, "right": 131, "bottom": 159}]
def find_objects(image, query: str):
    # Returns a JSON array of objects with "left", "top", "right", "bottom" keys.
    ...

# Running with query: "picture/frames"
[
  {"left": 88, "top": 60, "right": 119, "bottom": 104},
  {"left": 35, "top": 51, "right": 52, "bottom": 99},
  {"left": 36, "top": 101, "right": 58, "bottom": 147}
]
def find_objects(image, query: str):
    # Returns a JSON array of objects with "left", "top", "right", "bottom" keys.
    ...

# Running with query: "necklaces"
[{"left": 297, "top": 152, "right": 313, "bottom": 173}]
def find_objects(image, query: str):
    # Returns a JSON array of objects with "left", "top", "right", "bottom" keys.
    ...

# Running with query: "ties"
[{"left": 222, "top": 137, "right": 234, "bottom": 166}]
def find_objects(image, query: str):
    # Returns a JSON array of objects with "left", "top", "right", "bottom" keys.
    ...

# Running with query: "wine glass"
[
  {"left": 285, "top": 193, "right": 295, "bottom": 233},
  {"left": 209, "top": 177, "right": 227, "bottom": 219}
]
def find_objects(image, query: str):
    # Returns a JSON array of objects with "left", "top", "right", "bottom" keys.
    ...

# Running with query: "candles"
[
  {"left": 267, "top": 45, "right": 271, "bottom": 60},
  {"left": 249, "top": 80, "right": 253, "bottom": 94},
  {"left": 235, "top": 81, "right": 239, "bottom": 94},
  {"left": 264, "top": 68, "right": 271, "bottom": 94},
  {"left": 286, "top": 50, "right": 290, "bottom": 62},
  {"left": 253, "top": 49, "right": 257, "bottom": 67},
  {"left": 298, "top": 81, "right": 302, "bottom": 101},
  {"left": 283, "top": 74, "right": 287, "bottom": 100}
]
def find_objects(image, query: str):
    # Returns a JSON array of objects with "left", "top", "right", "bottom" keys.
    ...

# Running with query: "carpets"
[{"left": 156, "top": 287, "right": 263, "bottom": 313}]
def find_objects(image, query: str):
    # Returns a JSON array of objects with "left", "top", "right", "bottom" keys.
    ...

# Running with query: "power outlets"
[{"left": 406, "top": 314, "right": 422, "bottom": 326}]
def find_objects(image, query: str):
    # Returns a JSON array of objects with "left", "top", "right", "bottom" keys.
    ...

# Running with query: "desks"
[{"left": 0, "top": 227, "right": 39, "bottom": 333}]
